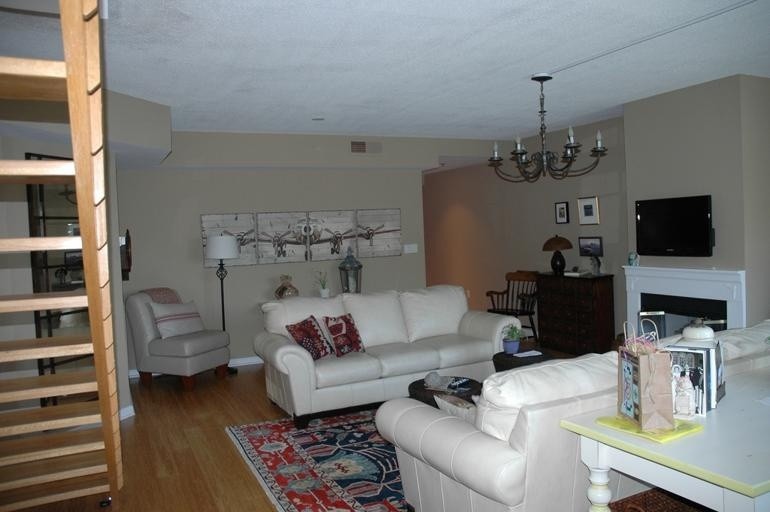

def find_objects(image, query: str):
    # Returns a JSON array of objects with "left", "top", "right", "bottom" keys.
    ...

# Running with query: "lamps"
[
  {"left": 206, "top": 236, "right": 241, "bottom": 375},
  {"left": 542, "top": 235, "right": 573, "bottom": 275},
  {"left": 485, "top": 73, "right": 607, "bottom": 182}
]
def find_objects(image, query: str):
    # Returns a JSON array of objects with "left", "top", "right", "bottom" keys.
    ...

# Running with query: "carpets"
[{"left": 221, "top": 410, "right": 413, "bottom": 512}]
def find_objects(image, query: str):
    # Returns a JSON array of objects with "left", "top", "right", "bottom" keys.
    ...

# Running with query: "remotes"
[{"left": 450, "top": 378, "right": 470, "bottom": 388}]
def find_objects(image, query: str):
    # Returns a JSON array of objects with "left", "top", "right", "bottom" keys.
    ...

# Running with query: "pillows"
[
  {"left": 323, "top": 313, "right": 366, "bottom": 357},
  {"left": 284, "top": 314, "right": 335, "bottom": 362},
  {"left": 149, "top": 301, "right": 206, "bottom": 339}
]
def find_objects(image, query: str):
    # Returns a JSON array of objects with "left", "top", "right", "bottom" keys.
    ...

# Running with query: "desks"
[{"left": 560, "top": 368, "right": 770, "bottom": 512}]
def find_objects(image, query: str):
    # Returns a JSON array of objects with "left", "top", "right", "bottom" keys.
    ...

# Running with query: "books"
[
  {"left": 597, "top": 414, "right": 703, "bottom": 445},
  {"left": 514, "top": 349, "right": 544, "bottom": 359},
  {"left": 563, "top": 269, "right": 589, "bottom": 278},
  {"left": 661, "top": 340, "right": 725, "bottom": 415}
]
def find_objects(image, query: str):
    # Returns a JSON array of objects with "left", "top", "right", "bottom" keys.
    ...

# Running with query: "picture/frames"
[
  {"left": 554, "top": 201, "right": 569, "bottom": 224},
  {"left": 578, "top": 237, "right": 603, "bottom": 257},
  {"left": 576, "top": 196, "right": 600, "bottom": 226}
]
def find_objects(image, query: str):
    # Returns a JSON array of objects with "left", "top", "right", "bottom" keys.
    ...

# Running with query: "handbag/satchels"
[{"left": 618, "top": 319, "right": 675, "bottom": 432}]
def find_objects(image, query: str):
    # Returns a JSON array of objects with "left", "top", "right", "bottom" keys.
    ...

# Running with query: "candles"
[
  {"left": 565, "top": 138, "right": 571, "bottom": 154},
  {"left": 514, "top": 133, "right": 521, "bottom": 151},
  {"left": 568, "top": 124, "right": 574, "bottom": 144},
  {"left": 595, "top": 128, "right": 603, "bottom": 147},
  {"left": 491, "top": 140, "right": 499, "bottom": 158},
  {"left": 521, "top": 143, "right": 526, "bottom": 159}
]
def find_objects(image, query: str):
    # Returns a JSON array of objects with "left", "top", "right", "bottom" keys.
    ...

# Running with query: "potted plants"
[
  {"left": 502, "top": 324, "right": 520, "bottom": 357},
  {"left": 314, "top": 272, "right": 330, "bottom": 297}
]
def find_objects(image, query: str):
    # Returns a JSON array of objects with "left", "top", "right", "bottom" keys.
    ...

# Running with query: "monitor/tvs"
[{"left": 635, "top": 195, "right": 712, "bottom": 257}]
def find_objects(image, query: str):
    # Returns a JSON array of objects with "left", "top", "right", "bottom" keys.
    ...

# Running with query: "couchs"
[
  {"left": 376, "top": 316, "right": 770, "bottom": 512},
  {"left": 253, "top": 284, "right": 521, "bottom": 429},
  {"left": 126, "top": 287, "right": 229, "bottom": 391}
]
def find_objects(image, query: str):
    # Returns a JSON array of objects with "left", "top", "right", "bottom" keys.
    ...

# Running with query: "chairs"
[{"left": 485, "top": 270, "right": 538, "bottom": 343}]
